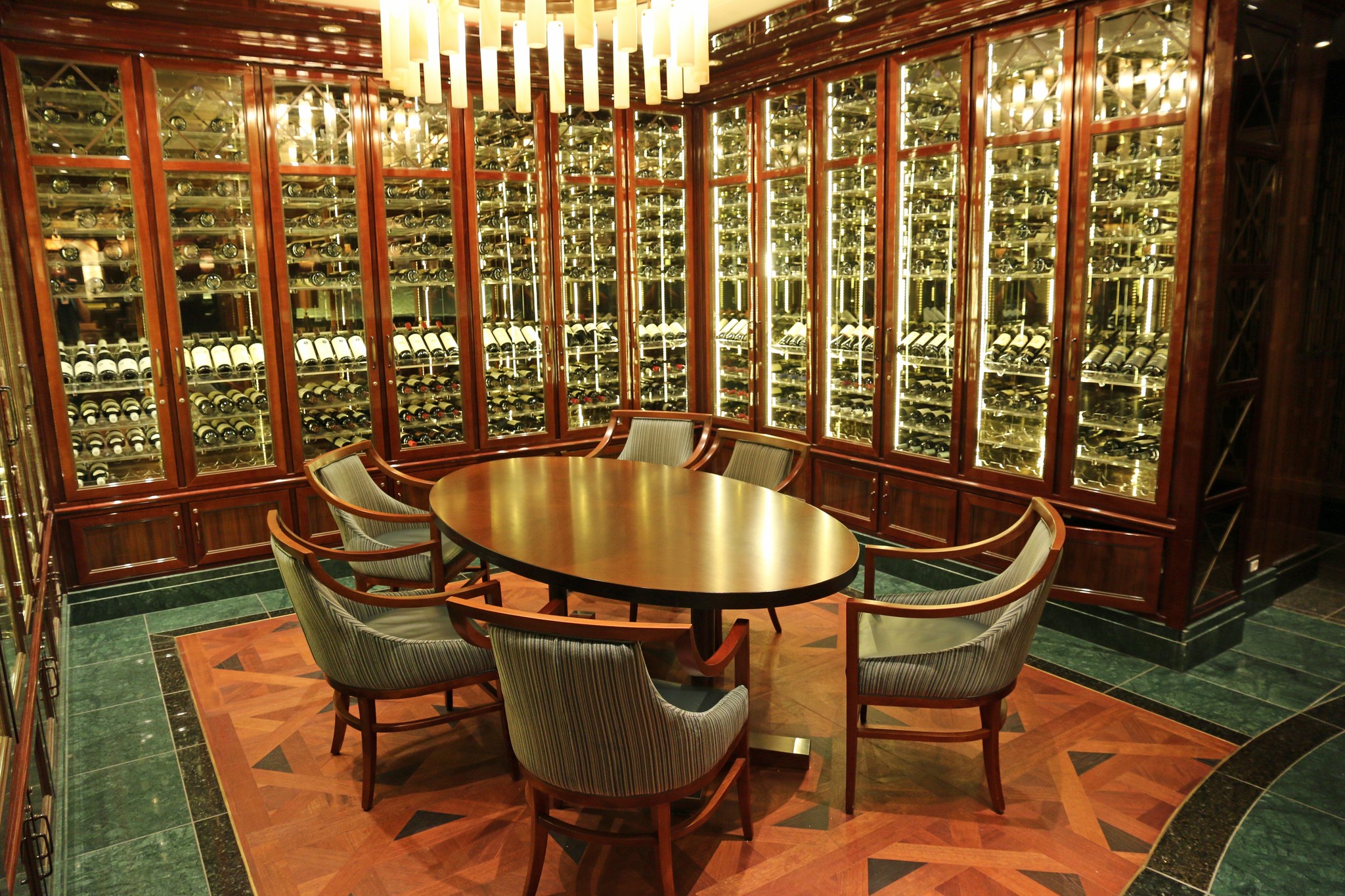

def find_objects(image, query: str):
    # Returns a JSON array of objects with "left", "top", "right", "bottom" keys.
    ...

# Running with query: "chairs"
[
  {"left": 584, "top": 409, "right": 714, "bottom": 469},
  {"left": 303, "top": 440, "right": 492, "bottom": 606},
  {"left": 445, "top": 596, "right": 757, "bottom": 896},
  {"left": 265, "top": 509, "right": 526, "bottom": 813},
  {"left": 843, "top": 492, "right": 1067, "bottom": 817},
  {"left": 687, "top": 427, "right": 811, "bottom": 495}
]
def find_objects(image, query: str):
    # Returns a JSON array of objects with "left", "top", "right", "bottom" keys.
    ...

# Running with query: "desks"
[{"left": 429, "top": 455, "right": 861, "bottom": 835}]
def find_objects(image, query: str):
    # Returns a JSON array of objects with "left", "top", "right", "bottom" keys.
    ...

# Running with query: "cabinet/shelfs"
[{"left": 0, "top": 0, "right": 1345, "bottom": 896}]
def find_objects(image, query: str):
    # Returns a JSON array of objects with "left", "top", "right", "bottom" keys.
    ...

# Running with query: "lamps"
[
  {"left": 379, "top": 0, "right": 712, "bottom": 113},
  {"left": 237, "top": 90, "right": 425, "bottom": 168},
  {"left": 990, "top": 52, "right": 1191, "bottom": 164}
]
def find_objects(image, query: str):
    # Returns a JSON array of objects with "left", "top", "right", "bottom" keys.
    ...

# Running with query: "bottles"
[{"left": 0, "top": 0, "right": 1188, "bottom": 896}]
